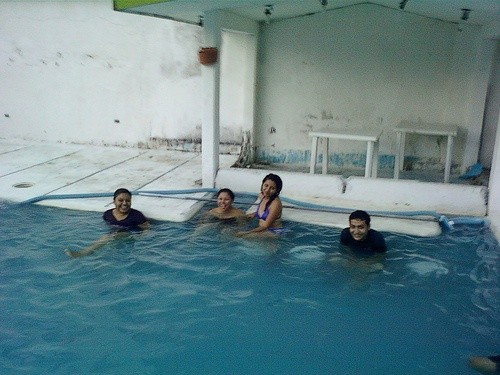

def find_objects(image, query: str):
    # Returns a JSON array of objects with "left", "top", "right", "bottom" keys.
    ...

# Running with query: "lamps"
[
  {"left": 263, "top": 4, "right": 273, "bottom": 16},
  {"left": 196, "top": 15, "right": 204, "bottom": 26},
  {"left": 461, "top": 7, "right": 474, "bottom": 20},
  {"left": 400, "top": 0, "right": 411, "bottom": 9},
  {"left": 319, "top": 0, "right": 331, "bottom": 7}
]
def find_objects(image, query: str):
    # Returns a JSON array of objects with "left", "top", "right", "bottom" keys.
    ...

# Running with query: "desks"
[
  {"left": 309, "top": 124, "right": 383, "bottom": 178},
  {"left": 394, "top": 119, "right": 460, "bottom": 182}
]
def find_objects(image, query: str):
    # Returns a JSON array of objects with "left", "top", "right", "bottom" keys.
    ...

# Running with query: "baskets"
[{"left": 199, "top": 48, "right": 217, "bottom": 64}]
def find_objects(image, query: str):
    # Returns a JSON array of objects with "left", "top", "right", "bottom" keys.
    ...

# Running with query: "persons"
[
  {"left": 103, "top": 188, "right": 150, "bottom": 227},
  {"left": 245, "top": 174, "right": 283, "bottom": 227},
  {"left": 341, "top": 210, "right": 387, "bottom": 253},
  {"left": 471, "top": 355, "right": 500, "bottom": 375},
  {"left": 209, "top": 188, "right": 241, "bottom": 219}
]
